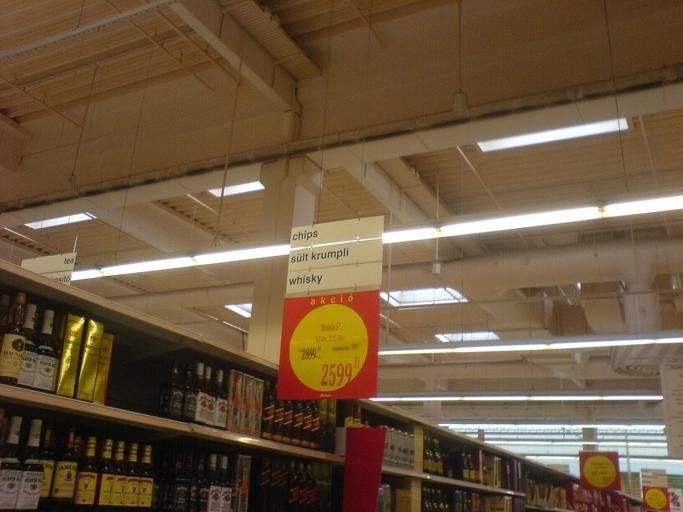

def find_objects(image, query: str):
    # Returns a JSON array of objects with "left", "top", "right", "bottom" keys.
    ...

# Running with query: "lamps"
[{"left": 37, "top": 2, "right": 682, "bottom": 470}]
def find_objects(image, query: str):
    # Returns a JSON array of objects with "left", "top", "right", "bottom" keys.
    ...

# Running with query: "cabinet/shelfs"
[{"left": 1, "top": 251, "right": 659, "bottom": 511}]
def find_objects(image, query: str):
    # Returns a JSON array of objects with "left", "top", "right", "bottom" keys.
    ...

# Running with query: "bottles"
[
  {"left": 0, "top": 363, "right": 320, "bottom": 511},
  {"left": 0, "top": 292, "right": 58, "bottom": 394},
  {"left": 359, "top": 418, "right": 481, "bottom": 511}
]
